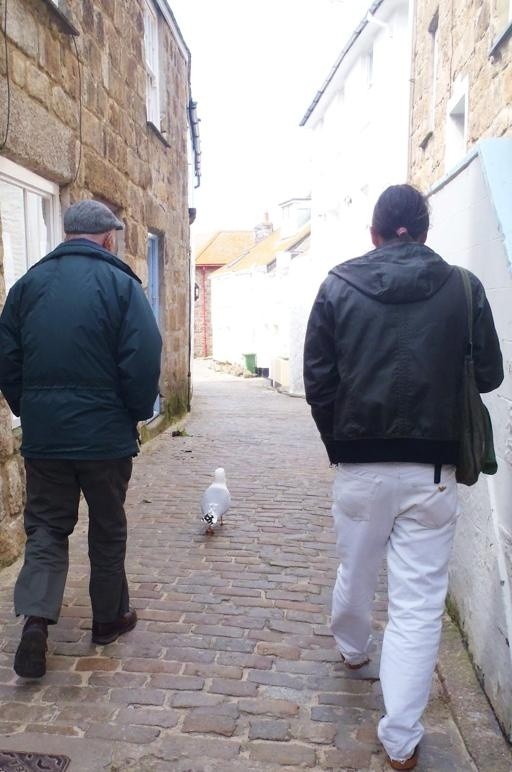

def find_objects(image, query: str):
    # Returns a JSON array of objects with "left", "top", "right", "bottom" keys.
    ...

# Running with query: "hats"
[{"left": 64, "top": 200, "right": 124, "bottom": 233}]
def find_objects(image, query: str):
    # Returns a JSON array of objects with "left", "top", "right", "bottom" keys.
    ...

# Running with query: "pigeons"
[{"left": 200, "top": 467, "right": 232, "bottom": 534}]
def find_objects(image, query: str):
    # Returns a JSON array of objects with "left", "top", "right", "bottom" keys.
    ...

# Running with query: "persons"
[
  {"left": 304, "top": 183, "right": 504, "bottom": 771},
  {"left": 0, "top": 199, "right": 162, "bottom": 679}
]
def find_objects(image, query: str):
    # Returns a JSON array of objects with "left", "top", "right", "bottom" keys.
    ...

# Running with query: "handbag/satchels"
[{"left": 455, "top": 356, "right": 497, "bottom": 486}]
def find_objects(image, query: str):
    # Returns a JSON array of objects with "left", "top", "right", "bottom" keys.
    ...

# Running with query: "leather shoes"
[
  {"left": 391, "top": 744, "right": 420, "bottom": 771},
  {"left": 339, "top": 652, "right": 370, "bottom": 669},
  {"left": 92, "top": 610, "right": 137, "bottom": 644},
  {"left": 14, "top": 616, "right": 48, "bottom": 678}
]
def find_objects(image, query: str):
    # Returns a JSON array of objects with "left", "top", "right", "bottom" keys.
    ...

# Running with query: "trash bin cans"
[{"left": 243, "top": 353, "right": 257, "bottom": 373}]
